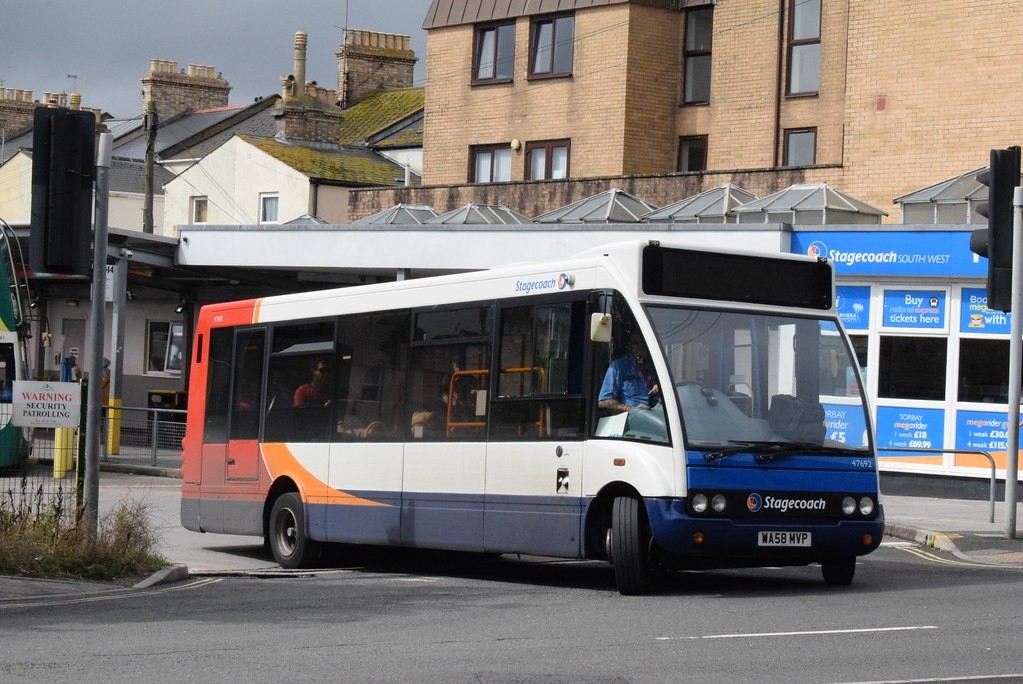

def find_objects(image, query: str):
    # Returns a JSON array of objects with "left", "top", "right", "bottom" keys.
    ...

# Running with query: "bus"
[
  {"left": 178, "top": 235, "right": 886, "bottom": 599},
  {"left": 0, "top": 215, "right": 53, "bottom": 475}
]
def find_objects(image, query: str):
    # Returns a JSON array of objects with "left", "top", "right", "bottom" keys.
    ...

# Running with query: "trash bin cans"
[{"left": 148, "top": 390, "right": 186, "bottom": 450}]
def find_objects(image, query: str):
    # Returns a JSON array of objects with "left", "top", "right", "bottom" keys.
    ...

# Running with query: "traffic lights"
[{"left": 969, "top": 147, "right": 1021, "bottom": 312}]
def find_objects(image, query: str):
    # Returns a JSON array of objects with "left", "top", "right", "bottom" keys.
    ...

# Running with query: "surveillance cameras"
[{"left": 123, "top": 250, "right": 133, "bottom": 259}]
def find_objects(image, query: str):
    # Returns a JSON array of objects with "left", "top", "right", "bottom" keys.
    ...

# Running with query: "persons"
[
  {"left": 597, "top": 329, "right": 660, "bottom": 413},
  {"left": 291, "top": 360, "right": 345, "bottom": 432},
  {"left": 102, "top": 358, "right": 111, "bottom": 416},
  {"left": 70, "top": 356, "right": 82, "bottom": 382},
  {"left": 425, "top": 346, "right": 478, "bottom": 430}
]
